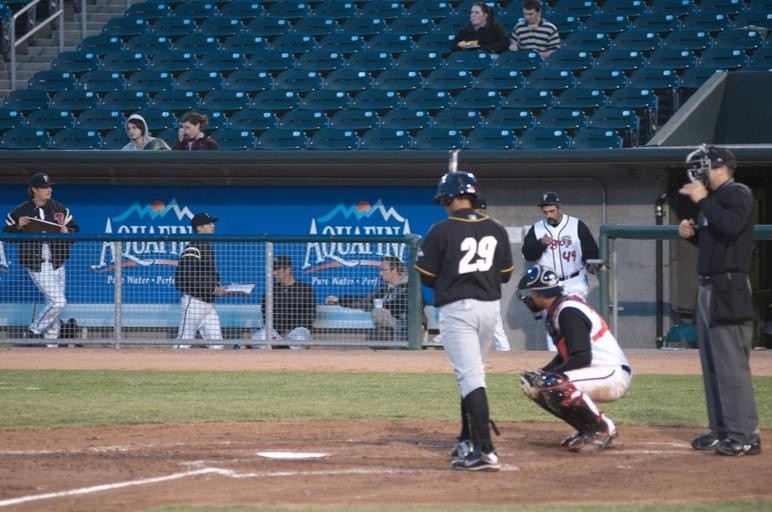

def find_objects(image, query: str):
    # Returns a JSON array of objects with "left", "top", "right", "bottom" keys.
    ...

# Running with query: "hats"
[
  {"left": 29, "top": 173, "right": 55, "bottom": 187},
  {"left": 536, "top": 191, "right": 559, "bottom": 207},
  {"left": 274, "top": 253, "right": 291, "bottom": 271},
  {"left": 192, "top": 212, "right": 217, "bottom": 228}
]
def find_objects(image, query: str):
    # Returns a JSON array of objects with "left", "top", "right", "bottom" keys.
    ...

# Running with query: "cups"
[
  {"left": 82, "top": 327, "right": 88, "bottom": 339},
  {"left": 374, "top": 298, "right": 387, "bottom": 309}
]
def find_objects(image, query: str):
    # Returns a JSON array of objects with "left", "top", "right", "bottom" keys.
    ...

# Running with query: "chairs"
[
  {"left": 1, "top": 0, "right": 772, "bottom": 149},
  {"left": 655, "top": 323, "right": 699, "bottom": 349}
]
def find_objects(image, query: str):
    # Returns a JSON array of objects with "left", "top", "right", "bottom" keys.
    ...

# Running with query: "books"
[{"left": 224, "top": 282, "right": 256, "bottom": 297}]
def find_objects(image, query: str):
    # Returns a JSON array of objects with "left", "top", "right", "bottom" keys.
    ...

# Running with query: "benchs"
[{"left": 0, "top": 302, "right": 444, "bottom": 348}]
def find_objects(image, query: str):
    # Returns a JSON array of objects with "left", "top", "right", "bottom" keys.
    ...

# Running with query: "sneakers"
[
  {"left": 690, "top": 435, "right": 723, "bottom": 451},
  {"left": 559, "top": 413, "right": 617, "bottom": 454},
  {"left": 451, "top": 449, "right": 499, "bottom": 472},
  {"left": 714, "top": 438, "right": 761, "bottom": 457},
  {"left": 448, "top": 438, "right": 472, "bottom": 462}
]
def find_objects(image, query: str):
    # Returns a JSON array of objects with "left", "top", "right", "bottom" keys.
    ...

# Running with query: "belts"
[{"left": 559, "top": 271, "right": 579, "bottom": 281}]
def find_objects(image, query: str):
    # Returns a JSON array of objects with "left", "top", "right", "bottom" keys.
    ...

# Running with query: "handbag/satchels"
[{"left": 706, "top": 273, "right": 757, "bottom": 328}]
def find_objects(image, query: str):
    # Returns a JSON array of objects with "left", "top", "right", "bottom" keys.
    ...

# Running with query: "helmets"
[
  {"left": 431, "top": 171, "right": 478, "bottom": 202},
  {"left": 514, "top": 265, "right": 565, "bottom": 296}
]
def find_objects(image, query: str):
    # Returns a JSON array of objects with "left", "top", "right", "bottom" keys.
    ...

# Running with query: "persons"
[
  {"left": 21, "top": 214, "right": 66, "bottom": 234},
  {"left": 172, "top": 211, "right": 231, "bottom": 352},
  {"left": 324, "top": 257, "right": 429, "bottom": 350},
  {"left": 678, "top": 144, "right": 763, "bottom": 458},
  {"left": 172, "top": 111, "right": 219, "bottom": 150},
  {"left": 508, "top": 0, "right": 561, "bottom": 60},
  {"left": 119, "top": 112, "right": 171, "bottom": 151},
  {"left": 1, "top": 172, "right": 80, "bottom": 348},
  {"left": 249, "top": 255, "right": 318, "bottom": 350},
  {"left": 450, "top": 1, "right": 511, "bottom": 54}
]
